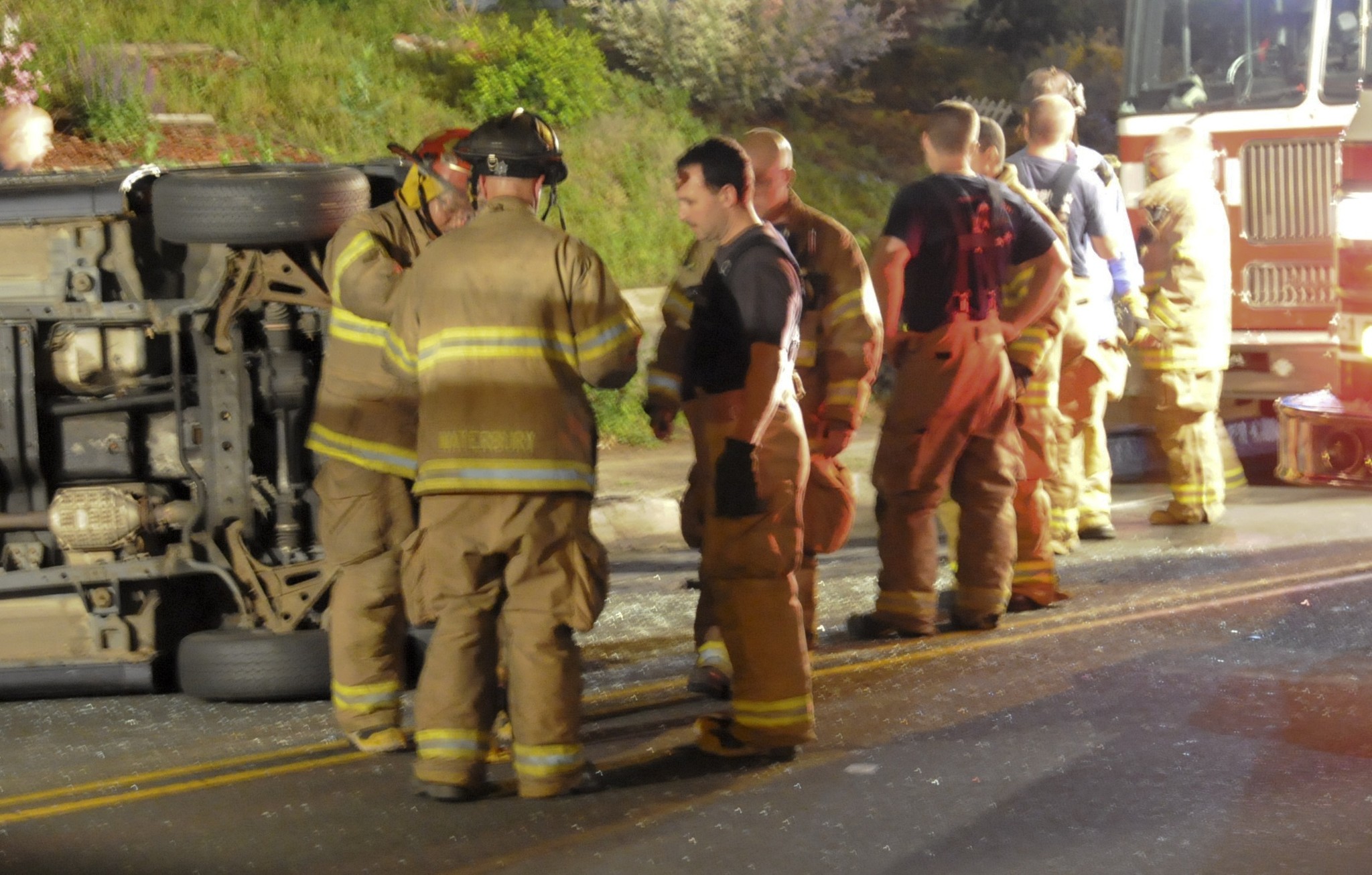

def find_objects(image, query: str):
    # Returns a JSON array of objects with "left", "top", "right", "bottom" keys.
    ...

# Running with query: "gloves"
[{"left": 715, "top": 438, "right": 760, "bottom": 521}]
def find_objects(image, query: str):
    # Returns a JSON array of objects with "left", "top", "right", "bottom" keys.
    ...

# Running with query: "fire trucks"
[{"left": 1109, "top": 0, "right": 1371, "bottom": 490}]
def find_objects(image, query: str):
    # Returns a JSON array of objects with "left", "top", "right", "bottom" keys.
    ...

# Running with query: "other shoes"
[
  {"left": 415, "top": 777, "right": 468, "bottom": 801},
  {"left": 694, "top": 714, "right": 801, "bottom": 764},
  {"left": 347, "top": 723, "right": 409, "bottom": 750},
  {"left": 1049, "top": 530, "right": 1082, "bottom": 554},
  {"left": 940, "top": 590, "right": 998, "bottom": 630},
  {"left": 1150, "top": 507, "right": 1205, "bottom": 526},
  {"left": 687, "top": 664, "right": 731, "bottom": 699},
  {"left": 1076, "top": 513, "right": 1115, "bottom": 538},
  {"left": 1008, "top": 591, "right": 1042, "bottom": 613},
  {"left": 847, "top": 609, "right": 927, "bottom": 639},
  {"left": 486, "top": 710, "right": 511, "bottom": 761}
]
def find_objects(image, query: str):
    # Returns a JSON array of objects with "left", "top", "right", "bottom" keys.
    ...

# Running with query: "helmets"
[
  {"left": 453, "top": 109, "right": 563, "bottom": 165},
  {"left": 388, "top": 128, "right": 476, "bottom": 204}
]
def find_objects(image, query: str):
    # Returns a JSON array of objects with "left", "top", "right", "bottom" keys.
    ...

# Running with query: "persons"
[
  {"left": 1007, "top": 66, "right": 1152, "bottom": 554},
  {"left": 942, "top": 118, "right": 1075, "bottom": 612},
  {"left": 643, "top": 127, "right": 883, "bottom": 698},
  {"left": 845, "top": 98, "right": 1070, "bottom": 638},
  {"left": 380, "top": 103, "right": 641, "bottom": 800},
  {"left": 1139, "top": 130, "right": 1250, "bottom": 525},
  {"left": 674, "top": 134, "right": 817, "bottom": 759},
  {"left": 304, "top": 127, "right": 512, "bottom": 764}
]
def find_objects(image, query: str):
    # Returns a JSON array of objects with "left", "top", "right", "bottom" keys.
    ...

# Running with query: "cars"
[{"left": 1, "top": 152, "right": 415, "bottom": 704}]
